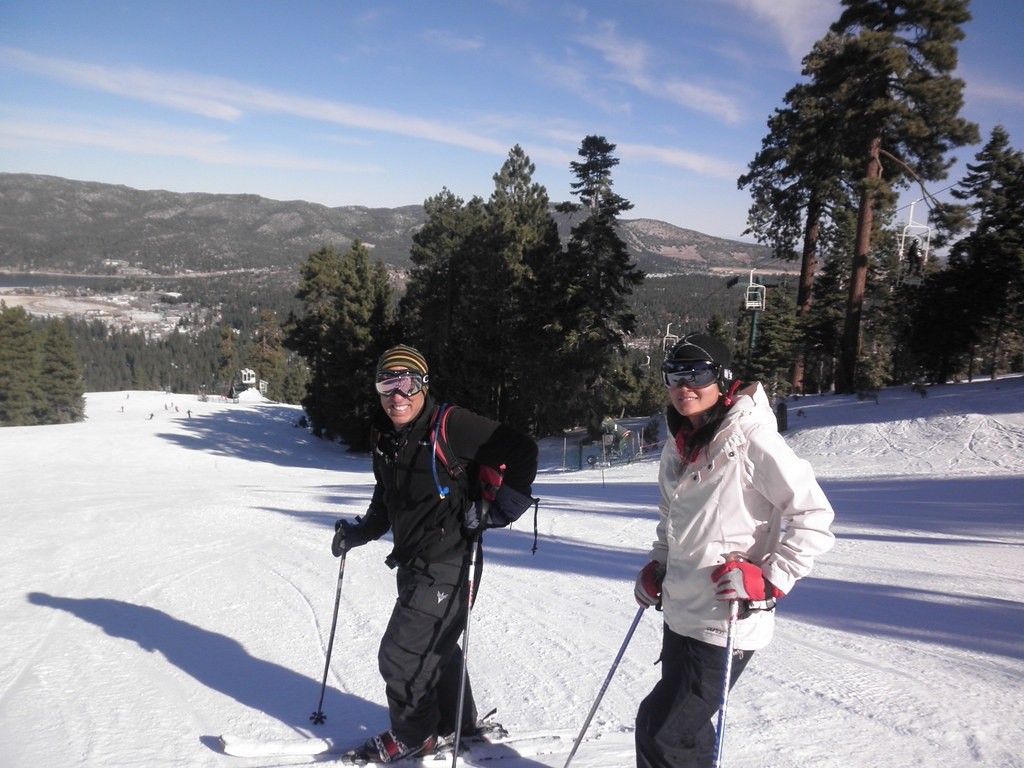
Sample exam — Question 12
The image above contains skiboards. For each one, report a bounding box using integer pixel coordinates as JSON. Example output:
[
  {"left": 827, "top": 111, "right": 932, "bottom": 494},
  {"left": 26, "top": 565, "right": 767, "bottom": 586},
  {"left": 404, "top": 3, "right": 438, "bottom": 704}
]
[{"left": 342, "top": 721, "right": 506, "bottom": 765}]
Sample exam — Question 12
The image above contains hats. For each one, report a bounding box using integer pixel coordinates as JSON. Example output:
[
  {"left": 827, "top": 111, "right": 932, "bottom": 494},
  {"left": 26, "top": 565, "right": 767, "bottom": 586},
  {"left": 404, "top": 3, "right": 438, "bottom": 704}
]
[
  {"left": 375, "top": 343, "right": 429, "bottom": 398},
  {"left": 668, "top": 332, "right": 732, "bottom": 398}
]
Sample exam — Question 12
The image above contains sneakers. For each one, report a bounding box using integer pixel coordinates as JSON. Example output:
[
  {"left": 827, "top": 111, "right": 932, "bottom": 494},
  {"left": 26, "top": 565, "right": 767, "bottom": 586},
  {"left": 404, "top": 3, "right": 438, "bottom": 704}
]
[
  {"left": 437, "top": 717, "right": 475, "bottom": 737},
  {"left": 364, "top": 729, "right": 437, "bottom": 762}
]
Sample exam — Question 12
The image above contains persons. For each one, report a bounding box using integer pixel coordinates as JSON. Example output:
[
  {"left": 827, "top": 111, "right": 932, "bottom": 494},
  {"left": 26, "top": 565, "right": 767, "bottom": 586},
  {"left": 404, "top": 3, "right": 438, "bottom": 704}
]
[
  {"left": 636, "top": 331, "right": 835, "bottom": 768},
  {"left": 120, "top": 394, "right": 193, "bottom": 419},
  {"left": 331, "top": 345, "right": 538, "bottom": 763},
  {"left": 298, "top": 415, "right": 310, "bottom": 431}
]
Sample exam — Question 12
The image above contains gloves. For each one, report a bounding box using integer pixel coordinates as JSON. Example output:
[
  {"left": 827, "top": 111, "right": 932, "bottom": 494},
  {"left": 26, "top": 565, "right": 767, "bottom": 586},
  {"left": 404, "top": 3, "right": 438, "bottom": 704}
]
[
  {"left": 483, "top": 505, "right": 507, "bottom": 530},
  {"left": 711, "top": 560, "right": 785, "bottom": 600},
  {"left": 332, "top": 516, "right": 370, "bottom": 557},
  {"left": 635, "top": 561, "right": 667, "bottom": 609}
]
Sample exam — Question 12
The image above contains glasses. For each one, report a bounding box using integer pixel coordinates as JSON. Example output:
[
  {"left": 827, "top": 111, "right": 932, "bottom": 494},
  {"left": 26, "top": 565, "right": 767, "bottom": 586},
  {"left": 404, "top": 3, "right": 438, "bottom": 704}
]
[
  {"left": 375, "top": 370, "right": 429, "bottom": 398},
  {"left": 661, "top": 361, "right": 734, "bottom": 390}
]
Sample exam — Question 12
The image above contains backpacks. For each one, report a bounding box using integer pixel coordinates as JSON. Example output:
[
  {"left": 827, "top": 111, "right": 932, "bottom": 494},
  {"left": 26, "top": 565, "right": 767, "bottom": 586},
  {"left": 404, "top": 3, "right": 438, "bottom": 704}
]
[{"left": 375, "top": 405, "right": 506, "bottom": 529}]
